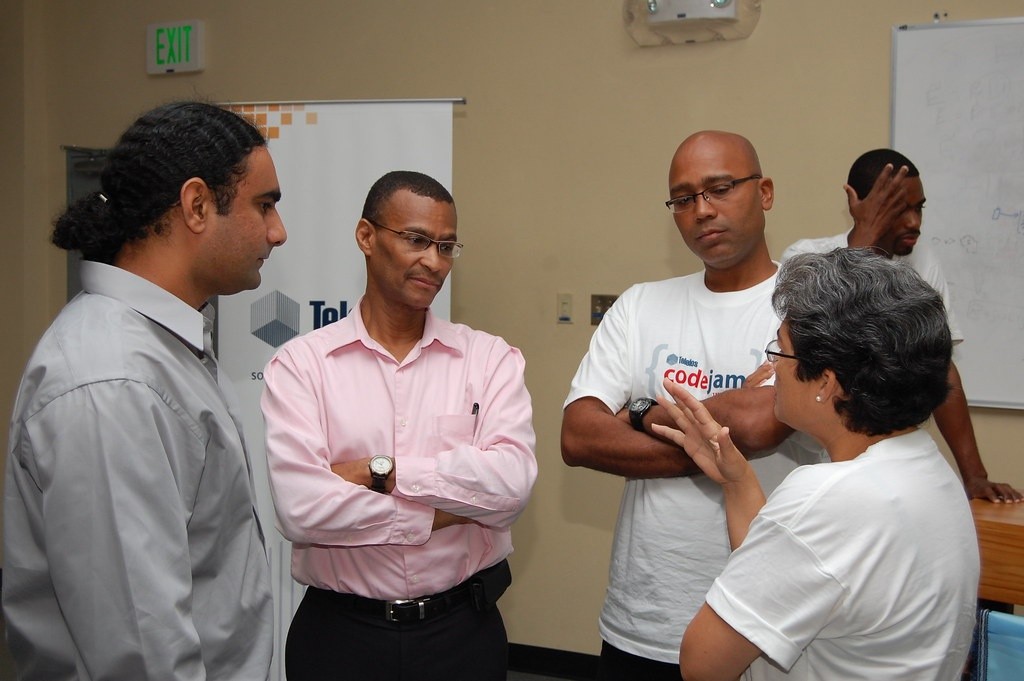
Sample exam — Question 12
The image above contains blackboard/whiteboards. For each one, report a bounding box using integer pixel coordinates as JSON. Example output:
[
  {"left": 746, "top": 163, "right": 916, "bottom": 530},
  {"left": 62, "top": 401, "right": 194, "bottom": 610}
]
[{"left": 887, "top": 18, "right": 1024, "bottom": 410}]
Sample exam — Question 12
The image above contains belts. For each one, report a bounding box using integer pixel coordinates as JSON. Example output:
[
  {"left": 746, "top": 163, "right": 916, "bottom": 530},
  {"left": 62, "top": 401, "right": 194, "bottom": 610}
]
[{"left": 310, "top": 579, "right": 477, "bottom": 623}]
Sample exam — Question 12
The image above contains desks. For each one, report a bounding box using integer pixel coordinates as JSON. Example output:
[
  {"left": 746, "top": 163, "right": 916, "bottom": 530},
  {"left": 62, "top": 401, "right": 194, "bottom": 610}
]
[{"left": 968, "top": 495, "right": 1024, "bottom": 616}]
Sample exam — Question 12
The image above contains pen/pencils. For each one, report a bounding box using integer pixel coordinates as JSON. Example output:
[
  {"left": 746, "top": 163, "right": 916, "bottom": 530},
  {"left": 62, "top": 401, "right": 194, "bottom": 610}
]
[{"left": 472, "top": 403, "right": 479, "bottom": 416}]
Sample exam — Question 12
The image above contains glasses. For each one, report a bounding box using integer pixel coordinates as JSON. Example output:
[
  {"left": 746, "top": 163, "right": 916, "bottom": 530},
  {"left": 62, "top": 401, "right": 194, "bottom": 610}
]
[
  {"left": 370, "top": 220, "right": 464, "bottom": 259},
  {"left": 665, "top": 175, "right": 762, "bottom": 214},
  {"left": 765, "top": 340, "right": 800, "bottom": 362}
]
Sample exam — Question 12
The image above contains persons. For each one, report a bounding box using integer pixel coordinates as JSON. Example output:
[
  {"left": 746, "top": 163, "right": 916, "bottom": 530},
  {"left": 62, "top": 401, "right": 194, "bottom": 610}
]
[
  {"left": 649, "top": 245, "right": 979, "bottom": 681},
  {"left": 777, "top": 147, "right": 1024, "bottom": 505},
  {"left": 263, "top": 169, "right": 537, "bottom": 681},
  {"left": 0, "top": 99, "right": 286, "bottom": 681},
  {"left": 558, "top": 132, "right": 828, "bottom": 680}
]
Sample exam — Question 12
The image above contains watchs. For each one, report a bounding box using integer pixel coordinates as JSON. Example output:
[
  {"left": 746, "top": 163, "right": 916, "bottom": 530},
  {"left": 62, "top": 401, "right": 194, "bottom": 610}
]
[
  {"left": 628, "top": 398, "right": 659, "bottom": 434},
  {"left": 367, "top": 454, "right": 394, "bottom": 493}
]
